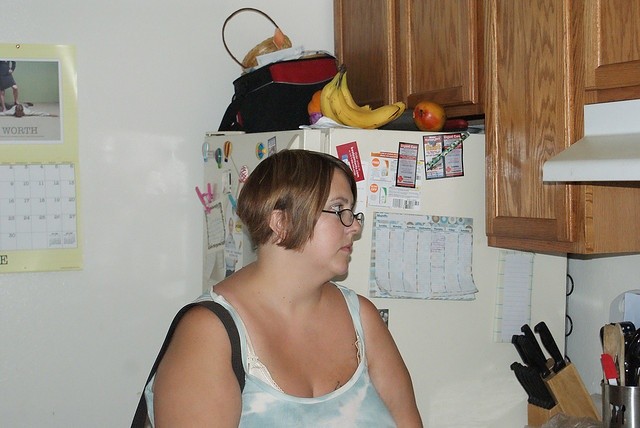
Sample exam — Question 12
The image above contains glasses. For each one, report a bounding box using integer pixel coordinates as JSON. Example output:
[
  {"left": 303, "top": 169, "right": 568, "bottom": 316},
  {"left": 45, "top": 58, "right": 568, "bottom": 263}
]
[{"left": 319, "top": 208, "right": 364, "bottom": 226}]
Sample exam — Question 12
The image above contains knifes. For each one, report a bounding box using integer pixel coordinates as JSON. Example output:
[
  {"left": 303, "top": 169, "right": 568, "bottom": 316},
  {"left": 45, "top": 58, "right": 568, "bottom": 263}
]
[{"left": 510, "top": 321, "right": 602, "bottom": 428}]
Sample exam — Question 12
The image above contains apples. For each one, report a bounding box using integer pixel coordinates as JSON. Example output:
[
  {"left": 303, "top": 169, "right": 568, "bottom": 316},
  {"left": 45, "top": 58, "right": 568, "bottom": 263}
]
[{"left": 413, "top": 101, "right": 446, "bottom": 130}]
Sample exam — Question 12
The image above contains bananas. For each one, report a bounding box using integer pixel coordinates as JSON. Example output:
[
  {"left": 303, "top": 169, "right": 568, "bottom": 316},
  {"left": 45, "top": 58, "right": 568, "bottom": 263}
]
[{"left": 321, "top": 64, "right": 405, "bottom": 129}]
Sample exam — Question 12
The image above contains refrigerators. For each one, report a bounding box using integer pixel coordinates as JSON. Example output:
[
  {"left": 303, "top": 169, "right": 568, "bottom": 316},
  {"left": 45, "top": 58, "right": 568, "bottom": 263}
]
[{"left": 201, "top": 131, "right": 567, "bottom": 428}]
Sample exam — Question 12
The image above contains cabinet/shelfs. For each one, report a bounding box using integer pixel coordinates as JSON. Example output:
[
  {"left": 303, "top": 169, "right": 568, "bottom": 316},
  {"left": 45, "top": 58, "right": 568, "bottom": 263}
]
[
  {"left": 584, "top": 0, "right": 640, "bottom": 105},
  {"left": 333, "top": 0, "right": 484, "bottom": 117},
  {"left": 484, "top": 0, "right": 640, "bottom": 255}
]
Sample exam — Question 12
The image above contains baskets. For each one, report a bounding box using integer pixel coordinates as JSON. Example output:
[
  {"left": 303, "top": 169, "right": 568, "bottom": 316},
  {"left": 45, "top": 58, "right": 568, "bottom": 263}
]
[{"left": 220, "top": 7, "right": 292, "bottom": 67}]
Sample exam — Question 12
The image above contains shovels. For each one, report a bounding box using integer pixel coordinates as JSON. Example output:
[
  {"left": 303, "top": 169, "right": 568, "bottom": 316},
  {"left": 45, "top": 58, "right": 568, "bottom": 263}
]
[
  {"left": 615, "top": 323, "right": 625, "bottom": 386},
  {"left": 603, "top": 323, "right": 620, "bottom": 384},
  {"left": 600, "top": 354, "right": 618, "bottom": 386},
  {"left": 618, "top": 322, "right": 636, "bottom": 385}
]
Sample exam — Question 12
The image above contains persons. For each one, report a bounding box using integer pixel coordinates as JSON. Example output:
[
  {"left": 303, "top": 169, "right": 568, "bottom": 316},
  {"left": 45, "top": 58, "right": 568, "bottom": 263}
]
[
  {"left": 0, "top": 61, "right": 20, "bottom": 112},
  {"left": 142, "top": 148, "right": 423, "bottom": 428}
]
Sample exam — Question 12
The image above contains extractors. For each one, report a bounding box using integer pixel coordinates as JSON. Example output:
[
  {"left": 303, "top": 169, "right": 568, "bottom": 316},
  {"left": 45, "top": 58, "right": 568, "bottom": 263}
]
[{"left": 542, "top": 99, "right": 640, "bottom": 182}]
